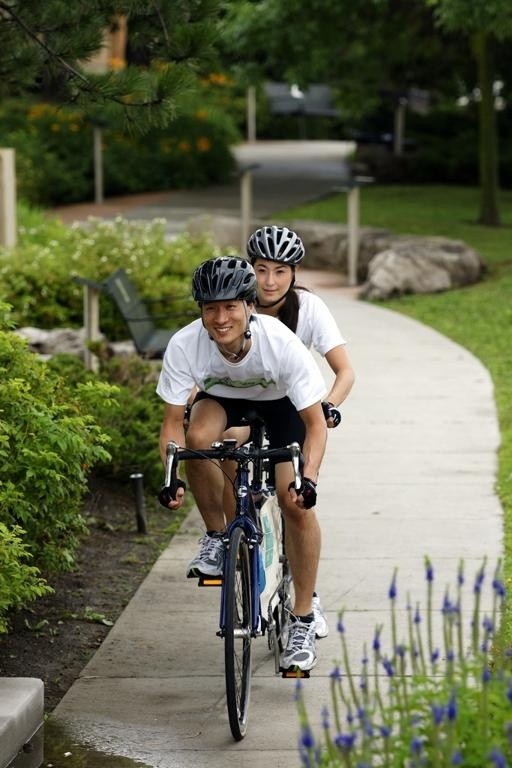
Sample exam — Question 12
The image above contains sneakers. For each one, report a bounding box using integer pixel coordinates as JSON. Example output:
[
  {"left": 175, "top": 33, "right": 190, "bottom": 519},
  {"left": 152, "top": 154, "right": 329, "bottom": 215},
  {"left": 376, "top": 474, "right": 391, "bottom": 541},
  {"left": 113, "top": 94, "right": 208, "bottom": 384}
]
[
  {"left": 186, "top": 535, "right": 225, "bottom": 577},
  {"left": 279, "top": 616, "right": 317, "bottom": 672},
  {"left": 313, "top": 594, "right": 329, "bottom": 638}
]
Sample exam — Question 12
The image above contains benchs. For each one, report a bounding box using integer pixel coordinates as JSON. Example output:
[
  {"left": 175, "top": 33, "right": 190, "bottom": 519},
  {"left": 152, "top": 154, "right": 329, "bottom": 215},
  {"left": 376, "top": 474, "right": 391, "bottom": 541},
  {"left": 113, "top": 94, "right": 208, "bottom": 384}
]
[{"left": 108, "top": 268, "right": 199, "bottom": 361}]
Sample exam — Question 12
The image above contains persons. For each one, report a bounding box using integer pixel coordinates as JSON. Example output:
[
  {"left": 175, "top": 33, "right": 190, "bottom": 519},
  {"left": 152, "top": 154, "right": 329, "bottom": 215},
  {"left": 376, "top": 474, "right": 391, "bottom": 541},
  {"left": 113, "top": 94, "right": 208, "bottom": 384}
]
[
  {"left": 221, "top": 226, "right": 355, "bottom": 641},
  {"left": 153, "top": 254, "right": 335, "bottom": 674}
]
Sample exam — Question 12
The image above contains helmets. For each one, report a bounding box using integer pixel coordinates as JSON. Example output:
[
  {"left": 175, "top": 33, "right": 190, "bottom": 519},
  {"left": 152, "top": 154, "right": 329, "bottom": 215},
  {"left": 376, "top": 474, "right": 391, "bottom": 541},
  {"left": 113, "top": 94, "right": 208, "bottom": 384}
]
[
  {"left": 192, "top": 256, "right": 256, "bottom": 301},
  {"left": 247, "top": 226, "right": 305, "bottom": 264}
]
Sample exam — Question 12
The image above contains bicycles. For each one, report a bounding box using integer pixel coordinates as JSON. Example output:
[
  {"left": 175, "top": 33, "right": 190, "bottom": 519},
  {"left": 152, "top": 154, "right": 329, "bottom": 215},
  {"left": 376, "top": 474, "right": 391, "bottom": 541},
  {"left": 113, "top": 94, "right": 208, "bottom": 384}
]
[{"left": 164, "top": 413, "right": 304, "bottom": 741}]
